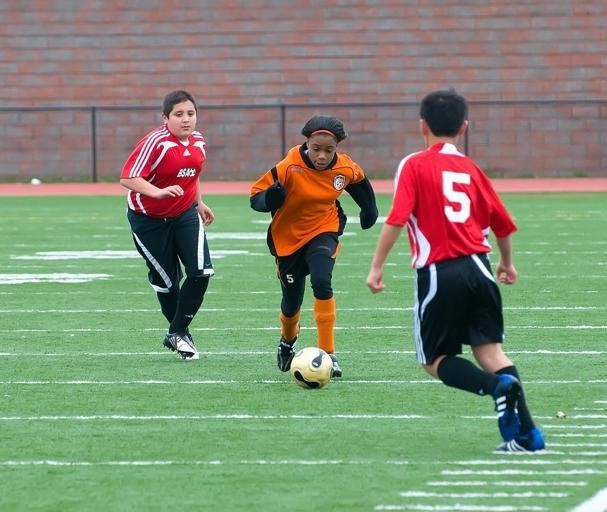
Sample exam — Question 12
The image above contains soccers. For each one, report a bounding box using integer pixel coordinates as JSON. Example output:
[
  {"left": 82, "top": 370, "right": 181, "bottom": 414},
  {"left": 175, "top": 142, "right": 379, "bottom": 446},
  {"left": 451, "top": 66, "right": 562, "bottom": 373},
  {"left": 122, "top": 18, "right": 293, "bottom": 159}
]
[
  {"left": 291, "top": 348, "right": 334, "bottom": 390},
  {"left": 29, "top": 178, "right": 42, "bottom": 185}
]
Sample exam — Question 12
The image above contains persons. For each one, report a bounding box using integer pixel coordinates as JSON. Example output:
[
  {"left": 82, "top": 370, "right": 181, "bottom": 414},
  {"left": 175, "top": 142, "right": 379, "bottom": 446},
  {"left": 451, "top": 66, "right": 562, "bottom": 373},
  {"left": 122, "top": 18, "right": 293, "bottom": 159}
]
[
  {"left": 249, "top": 114, "right": 379, "bottom": 377},
  {"left": 119, "top": 90, "right": 216, "bottom": 359},
  {"left": 366, "top": 90, "right": 545, "bottom": 453}
]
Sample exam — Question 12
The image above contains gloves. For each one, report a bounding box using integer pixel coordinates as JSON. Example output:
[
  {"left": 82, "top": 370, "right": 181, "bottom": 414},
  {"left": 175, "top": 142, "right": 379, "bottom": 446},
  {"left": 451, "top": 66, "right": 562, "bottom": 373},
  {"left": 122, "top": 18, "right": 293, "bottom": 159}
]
[
  {"left": 264, "top": 181, "right": 285, "bottom": 210},
  {"left": 359, "top": 206, "right": 378, "bottom": 229}
]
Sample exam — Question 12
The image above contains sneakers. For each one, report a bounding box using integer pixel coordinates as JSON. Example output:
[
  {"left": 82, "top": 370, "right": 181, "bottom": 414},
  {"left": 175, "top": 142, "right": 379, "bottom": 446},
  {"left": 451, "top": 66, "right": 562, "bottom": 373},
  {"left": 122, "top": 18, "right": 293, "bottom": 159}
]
[
  {"left": 492, "top": 374, "right": 545, "bottom": 455},
  {"left": 327, "top": 354, "right": 342, "bottom": 377},
  {"left": 277, "top": 322, "right": 300, "bottom": 372},
  {"left": 162, "top": 327, "right": 200, "bottom": 360}
]
